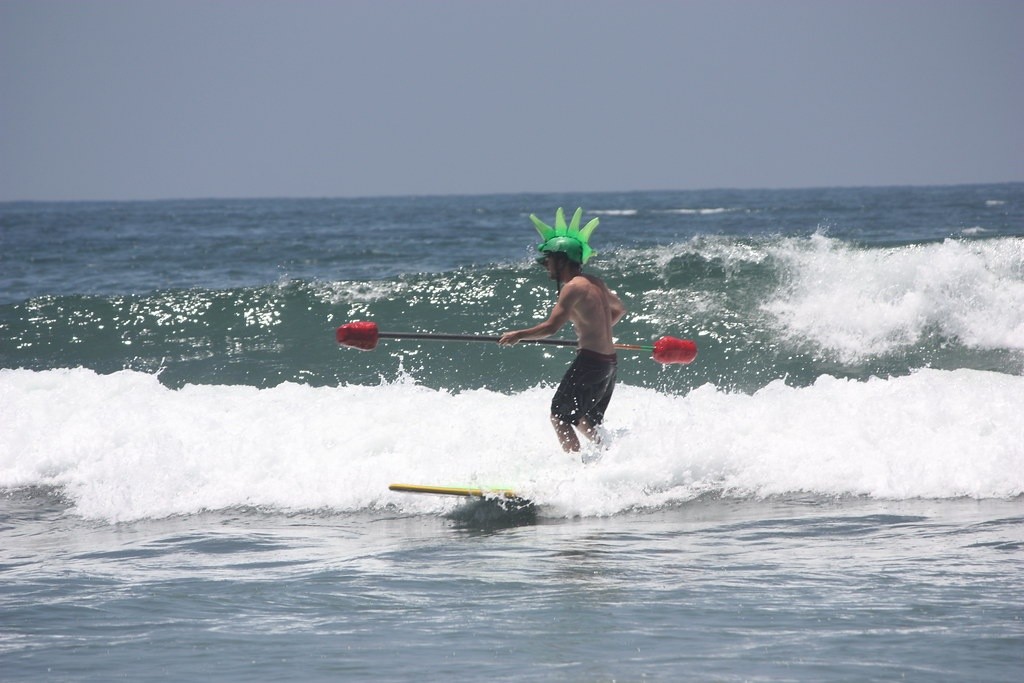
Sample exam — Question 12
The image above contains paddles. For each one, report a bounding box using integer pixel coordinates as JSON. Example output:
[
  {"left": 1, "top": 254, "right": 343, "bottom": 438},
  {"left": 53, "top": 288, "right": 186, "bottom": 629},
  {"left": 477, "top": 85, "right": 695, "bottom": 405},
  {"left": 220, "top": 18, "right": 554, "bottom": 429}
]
[{"left": 335, "top": 320, "right": 699, "bottom": 365}]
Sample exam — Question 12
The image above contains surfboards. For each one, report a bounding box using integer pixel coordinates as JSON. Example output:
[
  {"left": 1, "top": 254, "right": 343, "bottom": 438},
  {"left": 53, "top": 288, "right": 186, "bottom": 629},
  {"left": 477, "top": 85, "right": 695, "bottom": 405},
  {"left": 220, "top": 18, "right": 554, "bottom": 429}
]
[{"left": 388, "top": 482, "right": 519, "bottom": 499}]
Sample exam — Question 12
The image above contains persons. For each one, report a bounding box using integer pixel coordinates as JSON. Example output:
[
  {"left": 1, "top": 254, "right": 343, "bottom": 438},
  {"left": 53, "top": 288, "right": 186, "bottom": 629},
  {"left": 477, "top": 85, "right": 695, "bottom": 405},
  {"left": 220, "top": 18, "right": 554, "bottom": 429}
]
[{"left": 498, "top": 236, "right": 627, "bottom": 452}]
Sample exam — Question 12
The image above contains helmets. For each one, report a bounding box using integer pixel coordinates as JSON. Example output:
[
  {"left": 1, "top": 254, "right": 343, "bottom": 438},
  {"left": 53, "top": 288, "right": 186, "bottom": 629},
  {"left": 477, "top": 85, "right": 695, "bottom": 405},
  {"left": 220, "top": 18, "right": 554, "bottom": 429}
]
[{"left": 529, "top": 206, "right": 599, "bottom": 264}]
